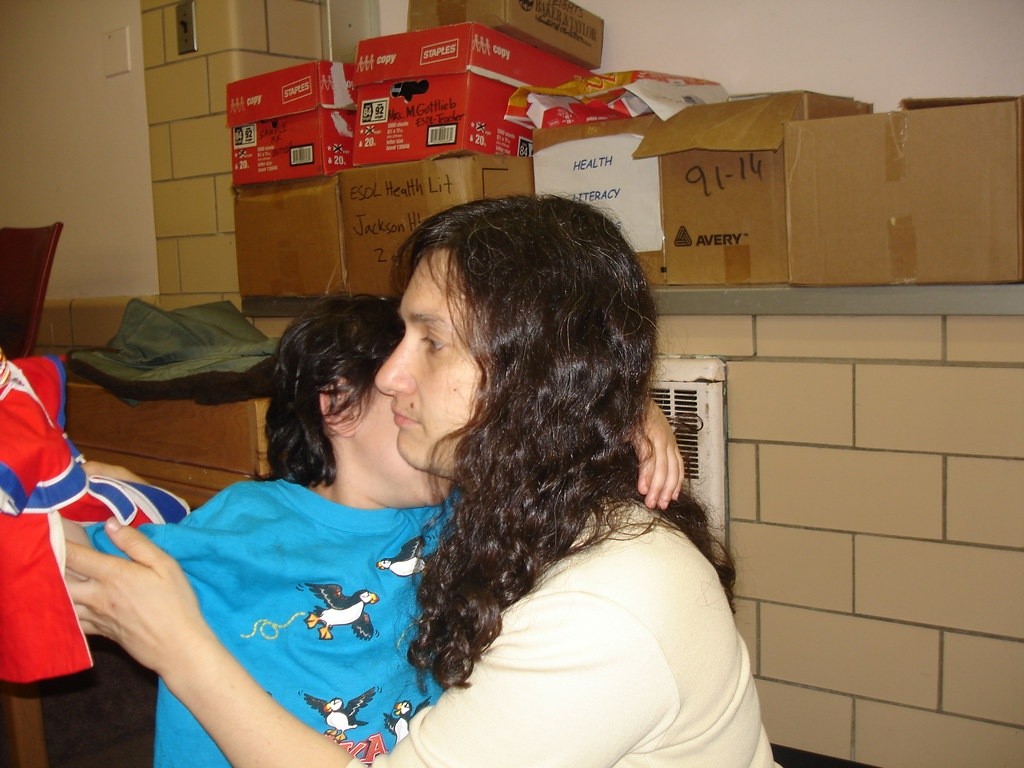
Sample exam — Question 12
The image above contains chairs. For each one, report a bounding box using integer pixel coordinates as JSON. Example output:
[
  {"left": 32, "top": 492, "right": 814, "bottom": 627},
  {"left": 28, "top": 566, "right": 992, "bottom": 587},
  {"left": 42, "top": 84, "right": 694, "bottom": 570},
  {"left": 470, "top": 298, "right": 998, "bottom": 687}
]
[{"left": 0, "top": 221, "right": 63, "bottom": 768}]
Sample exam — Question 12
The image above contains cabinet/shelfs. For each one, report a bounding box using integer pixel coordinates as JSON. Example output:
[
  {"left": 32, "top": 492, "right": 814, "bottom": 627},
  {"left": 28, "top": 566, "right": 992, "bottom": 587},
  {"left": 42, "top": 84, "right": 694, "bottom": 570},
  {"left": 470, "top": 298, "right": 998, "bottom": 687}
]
[{"left": 64, "top": 365, "right": 274, "bottom": 514}]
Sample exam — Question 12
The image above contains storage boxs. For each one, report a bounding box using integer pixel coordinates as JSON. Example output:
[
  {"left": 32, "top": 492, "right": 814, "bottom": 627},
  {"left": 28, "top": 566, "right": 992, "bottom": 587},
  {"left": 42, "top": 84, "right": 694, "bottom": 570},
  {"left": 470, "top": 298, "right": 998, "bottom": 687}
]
[{"left": 229, "top": 0, "right": 1024, "bottom": 299}]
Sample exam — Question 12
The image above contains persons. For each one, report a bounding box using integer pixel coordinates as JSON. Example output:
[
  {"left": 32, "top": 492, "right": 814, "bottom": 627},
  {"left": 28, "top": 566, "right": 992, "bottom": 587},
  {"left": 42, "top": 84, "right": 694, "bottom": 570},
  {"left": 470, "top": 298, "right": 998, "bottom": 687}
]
[
  {"left": 64, "top": 196, "right": 789, "bottom": 768},
  {"left": 64, "top": 294, "right": 687, "bottom": 768}
]
